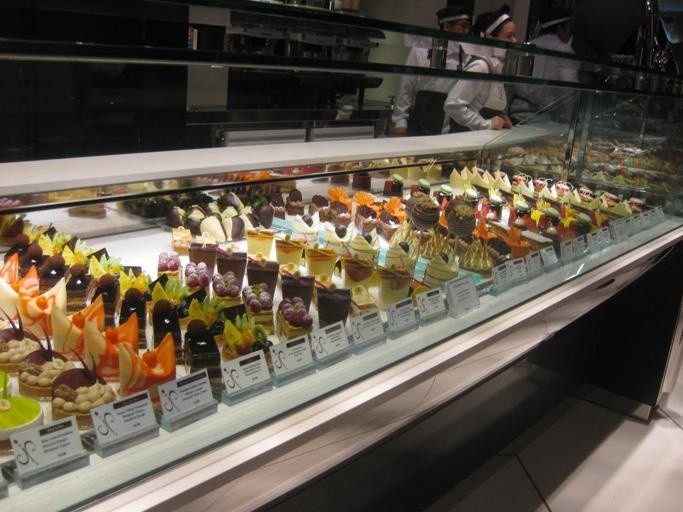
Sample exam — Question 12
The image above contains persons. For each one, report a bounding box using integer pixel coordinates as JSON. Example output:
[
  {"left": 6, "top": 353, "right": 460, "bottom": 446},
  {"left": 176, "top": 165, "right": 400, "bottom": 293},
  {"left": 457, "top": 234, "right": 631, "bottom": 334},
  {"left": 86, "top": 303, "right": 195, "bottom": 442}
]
[
  {"left": 438, "top": 3, "right": 524, "bottom": 131},
  {"left": 390, "top": 1, "right": 485, "bottom": 138},
  {"left": 505, "top": 7, "right": 582, "bottom": 126},
  {"left": 567, "top": 29, "right": 621, "bottom": 87}
]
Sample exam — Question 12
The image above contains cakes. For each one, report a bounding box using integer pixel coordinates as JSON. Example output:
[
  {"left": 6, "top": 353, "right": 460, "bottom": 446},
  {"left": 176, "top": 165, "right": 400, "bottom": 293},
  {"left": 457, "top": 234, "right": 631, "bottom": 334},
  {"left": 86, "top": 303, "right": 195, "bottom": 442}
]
[{"left": 502, "top": 138, "right": 680, "bottom": 195}]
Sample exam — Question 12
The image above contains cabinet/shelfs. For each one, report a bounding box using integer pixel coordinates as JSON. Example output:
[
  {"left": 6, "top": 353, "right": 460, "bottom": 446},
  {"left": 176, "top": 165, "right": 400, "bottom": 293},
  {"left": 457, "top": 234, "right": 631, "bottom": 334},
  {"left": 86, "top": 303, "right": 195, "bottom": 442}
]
[{"left": 0, "top": 0, "right": 683, "bottom": 512}]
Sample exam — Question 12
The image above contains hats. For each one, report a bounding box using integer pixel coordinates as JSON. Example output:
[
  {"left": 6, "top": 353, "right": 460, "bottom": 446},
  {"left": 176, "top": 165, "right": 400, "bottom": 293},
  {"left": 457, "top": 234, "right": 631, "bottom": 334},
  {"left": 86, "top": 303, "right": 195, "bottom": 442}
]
[
  {"left": 470, "top": 3, "right": 512, "bottom": 39},
  {"left": 538, "top": 7, "right": 570, "bottom": 29},
  {"left": 436, "top": 3, "right": 473, "bottom": 25}
]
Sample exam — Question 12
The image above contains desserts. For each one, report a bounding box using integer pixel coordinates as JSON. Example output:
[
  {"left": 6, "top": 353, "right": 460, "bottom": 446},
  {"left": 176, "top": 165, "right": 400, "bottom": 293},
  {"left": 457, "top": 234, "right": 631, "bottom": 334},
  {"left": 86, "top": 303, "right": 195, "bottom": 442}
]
[{"left": 1, "top": 160, "right": 645, "bottom": 440}]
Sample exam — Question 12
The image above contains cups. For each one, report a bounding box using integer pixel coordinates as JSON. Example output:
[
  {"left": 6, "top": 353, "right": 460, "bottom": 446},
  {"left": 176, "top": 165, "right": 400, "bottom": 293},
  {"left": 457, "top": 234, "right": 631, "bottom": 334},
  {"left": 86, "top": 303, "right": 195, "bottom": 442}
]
[{"left": 507, "top": 42, "right": 535, "bottom": 74}]
